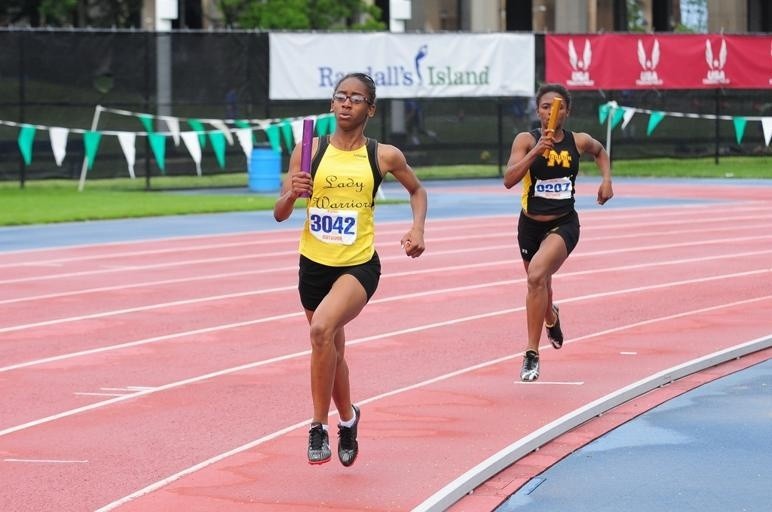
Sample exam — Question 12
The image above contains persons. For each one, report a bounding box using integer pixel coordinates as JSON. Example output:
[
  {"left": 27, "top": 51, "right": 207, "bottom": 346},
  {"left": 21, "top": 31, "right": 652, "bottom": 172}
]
[
  {"left": 272, "top": 70, "right": 429, "bottom": 467},
  {"left": 503, "top": 81, "right": 614, "bottom": 383}
]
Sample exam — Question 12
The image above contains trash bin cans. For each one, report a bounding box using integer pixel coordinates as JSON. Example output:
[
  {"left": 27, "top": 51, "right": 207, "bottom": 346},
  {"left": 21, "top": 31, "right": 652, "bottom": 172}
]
[{"left": 249, "top": 144, "right": 282, "bottom": 193}]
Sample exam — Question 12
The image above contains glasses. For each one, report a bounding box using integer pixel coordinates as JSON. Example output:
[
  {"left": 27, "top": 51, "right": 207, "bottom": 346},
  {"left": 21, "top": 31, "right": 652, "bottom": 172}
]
[{"left": 333, "top": 92, "right": 372, "bottom": 105}]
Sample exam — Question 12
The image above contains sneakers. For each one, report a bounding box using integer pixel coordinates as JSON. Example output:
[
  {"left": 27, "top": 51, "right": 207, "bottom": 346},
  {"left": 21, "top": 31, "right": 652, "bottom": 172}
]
[
  {"left": 544, "top": 304, "right": 564, "bottom": 349},
  {"left": 307, "top": 424, "right": 332, "bottom": 465},
  {"left": 337, "top": 404, "right": 361, "bottom": 467},
  {"left": 520, "top": 350, "right": 540, "bottom": 382}
]
